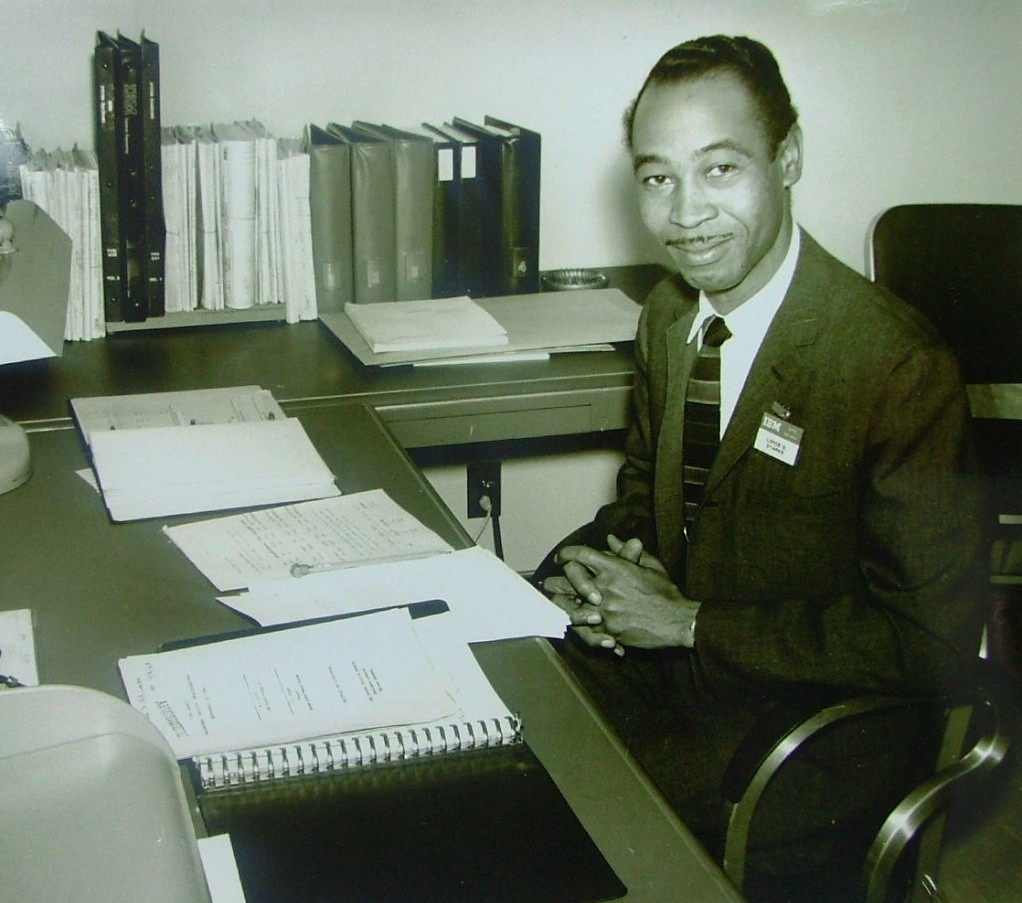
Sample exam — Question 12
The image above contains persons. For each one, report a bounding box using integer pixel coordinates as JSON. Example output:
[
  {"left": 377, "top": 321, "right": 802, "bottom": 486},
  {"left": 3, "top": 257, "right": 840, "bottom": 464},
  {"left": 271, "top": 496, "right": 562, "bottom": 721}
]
[{"left": 528, "top": 35, "right": 995, "bottom": 851}]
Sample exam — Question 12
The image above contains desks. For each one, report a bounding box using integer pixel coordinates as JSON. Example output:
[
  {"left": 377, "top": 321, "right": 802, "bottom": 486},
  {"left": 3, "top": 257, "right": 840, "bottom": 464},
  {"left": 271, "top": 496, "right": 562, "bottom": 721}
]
[
  {"left": 0, "top": 403, "right": 747, "bottom": 903},
  {"left": 1, "top": 262, "right": 673, "bottom": 448}
]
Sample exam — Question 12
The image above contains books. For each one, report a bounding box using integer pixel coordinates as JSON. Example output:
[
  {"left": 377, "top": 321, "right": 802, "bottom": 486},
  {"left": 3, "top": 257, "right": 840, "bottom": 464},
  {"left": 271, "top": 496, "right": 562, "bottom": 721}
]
[{"left": 0, "top": 29, "right": 648, "bottom": 795}]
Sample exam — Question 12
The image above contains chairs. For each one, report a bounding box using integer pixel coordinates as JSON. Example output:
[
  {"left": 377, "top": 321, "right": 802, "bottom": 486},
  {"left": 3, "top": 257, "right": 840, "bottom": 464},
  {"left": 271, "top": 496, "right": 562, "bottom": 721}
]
[{"left": 533, "top": 203, "right": 1022, "bottom": 903}]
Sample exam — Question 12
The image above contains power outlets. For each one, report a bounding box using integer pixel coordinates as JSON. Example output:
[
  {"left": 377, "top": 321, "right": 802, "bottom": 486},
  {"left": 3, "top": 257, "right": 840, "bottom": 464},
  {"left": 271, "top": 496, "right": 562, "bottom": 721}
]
[{"left": 467, "top": 460, "right": 501, "bottom": 519}]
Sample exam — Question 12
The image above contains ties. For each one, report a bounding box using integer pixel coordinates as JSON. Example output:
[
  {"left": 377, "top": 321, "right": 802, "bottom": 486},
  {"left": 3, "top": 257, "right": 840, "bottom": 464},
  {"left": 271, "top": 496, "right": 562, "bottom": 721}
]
[{"left": 682, "top": 314, "right": 732, "bottom": 553}]
